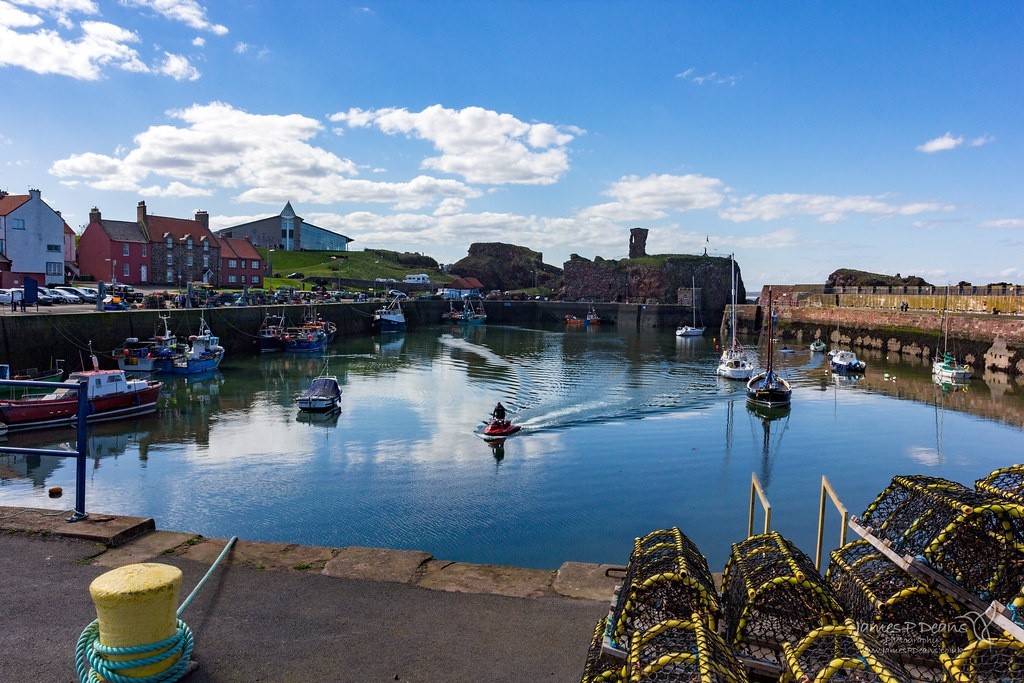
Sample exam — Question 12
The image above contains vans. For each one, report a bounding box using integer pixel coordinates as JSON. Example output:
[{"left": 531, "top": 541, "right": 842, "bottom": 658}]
[{"left": 436, "top": 292, "right": 450, "bottom": 300}]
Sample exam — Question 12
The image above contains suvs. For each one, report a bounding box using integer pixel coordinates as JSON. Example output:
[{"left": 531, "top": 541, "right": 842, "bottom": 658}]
[
  {"left": 105, "top": 284, "right": 144, "bottom": 303},
  {"left": 461, "top": 293, "right": 478, "bottom": 300}
]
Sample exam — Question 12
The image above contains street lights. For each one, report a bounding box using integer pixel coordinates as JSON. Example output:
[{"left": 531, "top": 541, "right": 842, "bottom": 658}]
[
  {"left": 268, "top": 247, "right": 275, "bottom": 291},
  {"left": 339, "top": 261, "right": 343, "bottom": 290},
  {"left": 828, "top": 310, "right": 843, "bottom": 359}
]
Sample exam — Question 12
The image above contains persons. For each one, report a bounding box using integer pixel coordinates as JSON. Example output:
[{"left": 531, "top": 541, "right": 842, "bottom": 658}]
[
  {"left": 492, "top": 402, "right": 505, "bottom": 426},
  {"left": 175, "top": 295, "right": 179, "bottom": 309},
  {"left": 900, "top": 301, "right": 909, "bottom": 311},
  {"left": 353, "top": 293, "right": 367, "bottom": 301}
]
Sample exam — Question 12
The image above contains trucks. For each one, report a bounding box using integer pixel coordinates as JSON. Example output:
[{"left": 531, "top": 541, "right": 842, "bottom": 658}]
[{"left": 403, "top": 275, "right": 429, "bottom": 284}]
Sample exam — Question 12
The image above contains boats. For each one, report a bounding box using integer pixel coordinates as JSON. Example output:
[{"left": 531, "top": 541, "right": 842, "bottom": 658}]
[
  {"left": 0, "top": 365, "right": 64, "bottom": 399},
  {"left": 0, "top": 369, "right": 163, "bottom": 433},
  {"left": 371, "top": 304, "right": 406, "bottom": 334},
  {"left": 565, "top": 313, "right": 602, "bottom": 327},
  {"left": 828, "top": 348, "right": 866, "bottom": 375},
  {"left": 152, "top": 317, "right": 225, "bottom": 373},
  {"left": 112, "top": 313, "right": 190, "bottom": 373},
  {"left": 810, "top": 340, "right": 826, "bottom": 352},
  {"left": 278, "top": 311, "right": 337, "bottom": 352},
  {"left": 256, "top": 314, "right": 300, "bottom": 353},
  {"left": 441, "top": 300, "right": 487, "bottom": 325},
  {"left": 294, "top": 360, "right": 343, "bottom": 409},
  {"left": 778, "top": 349, "right": 796, "bottom": 353}
]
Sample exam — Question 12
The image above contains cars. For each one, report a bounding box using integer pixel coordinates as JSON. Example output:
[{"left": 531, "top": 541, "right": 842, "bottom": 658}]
[
  {"left": 233, "top": 290, "right": 345, "bottom": 298},
  {"left": 387, "top": 279, "right": 399, "bottom": 282},
  {"left": 389, "top": 290, "right": 407, "bottom": 298},
  {"left": 286, "top": 273, "right": 305, "bottom": 279},
  {"left": 0, "top": 286, "right": 110, "bottom": 305},
  {"left": 479, "top": 294, "right": 512, "bottom": 301}
]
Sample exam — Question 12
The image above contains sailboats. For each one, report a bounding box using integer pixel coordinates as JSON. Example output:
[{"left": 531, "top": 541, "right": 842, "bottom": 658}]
[
  {"left": 746, "top": 286, "right": 792, "bottom": 408},
  {"left": 676, "top": 275, "right": 706, "bottom": 336},
  {"left": 932, "top": 282, "right": 975, "bottom": 379},
  {"left": 716, "top": 251, "right": 754, "bottom": 379}
]
